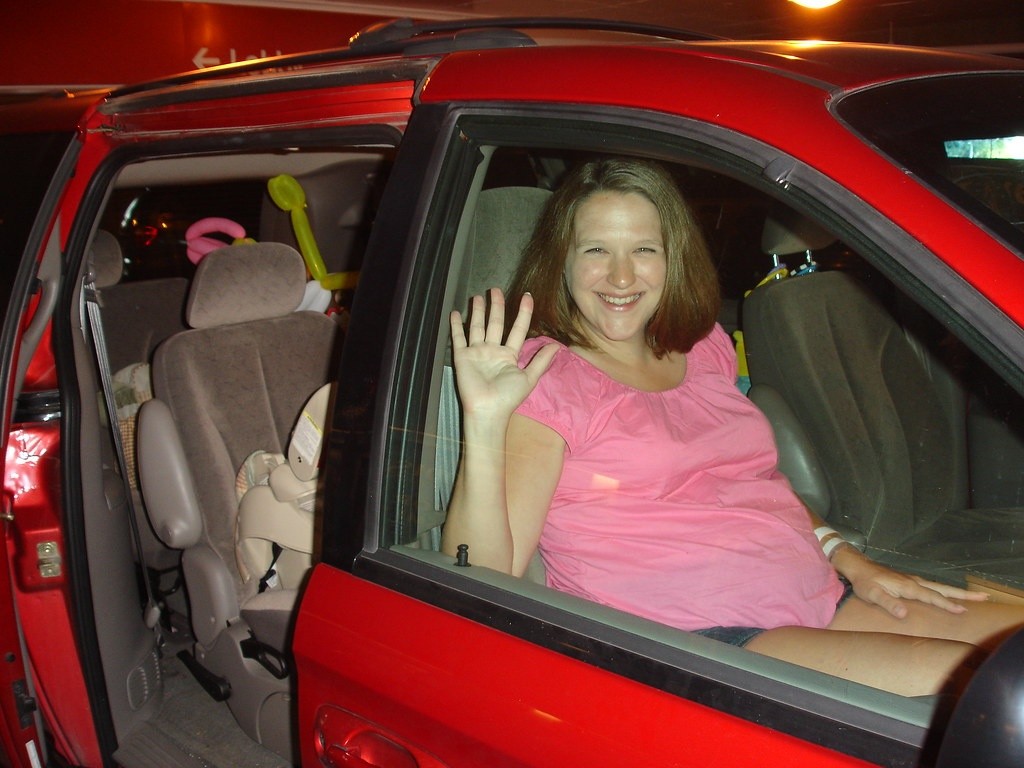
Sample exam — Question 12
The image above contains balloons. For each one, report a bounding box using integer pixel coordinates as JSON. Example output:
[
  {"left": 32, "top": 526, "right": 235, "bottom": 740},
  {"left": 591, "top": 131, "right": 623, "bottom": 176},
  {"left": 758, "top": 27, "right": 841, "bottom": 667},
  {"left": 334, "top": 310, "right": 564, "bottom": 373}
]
[{"left": 179, "top": 175, "right": 361, "bottom": 312}]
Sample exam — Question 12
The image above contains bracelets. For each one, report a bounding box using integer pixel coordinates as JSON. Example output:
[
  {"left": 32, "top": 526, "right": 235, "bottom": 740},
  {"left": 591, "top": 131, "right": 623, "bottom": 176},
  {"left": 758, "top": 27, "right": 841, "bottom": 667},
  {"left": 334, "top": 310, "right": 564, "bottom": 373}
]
[{"left": 813, "top": 526, "right": 854, "bottom": 558}]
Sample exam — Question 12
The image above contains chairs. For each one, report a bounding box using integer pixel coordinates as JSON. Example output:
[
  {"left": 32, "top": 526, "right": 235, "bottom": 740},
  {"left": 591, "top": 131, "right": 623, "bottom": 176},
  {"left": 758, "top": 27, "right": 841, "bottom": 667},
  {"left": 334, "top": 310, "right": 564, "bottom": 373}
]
[{"left": 92, "top": 161, "right": 1024, "bottom": 761}]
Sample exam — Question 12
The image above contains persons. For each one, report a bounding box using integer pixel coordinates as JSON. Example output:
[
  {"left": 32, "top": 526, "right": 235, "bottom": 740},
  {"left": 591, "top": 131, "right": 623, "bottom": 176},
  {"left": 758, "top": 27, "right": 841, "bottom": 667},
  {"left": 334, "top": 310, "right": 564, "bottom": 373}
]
[{"left": 437, "top": 159, "right": 1024, "bottom": 699}]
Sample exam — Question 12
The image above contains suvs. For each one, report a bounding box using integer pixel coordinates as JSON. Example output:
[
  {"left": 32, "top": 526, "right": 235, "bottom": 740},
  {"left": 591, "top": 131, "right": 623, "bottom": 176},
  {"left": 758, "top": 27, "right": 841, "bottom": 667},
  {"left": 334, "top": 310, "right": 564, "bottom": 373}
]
[{"left": 0, "top": 12, "right": 1024, "bottom": 767}]
